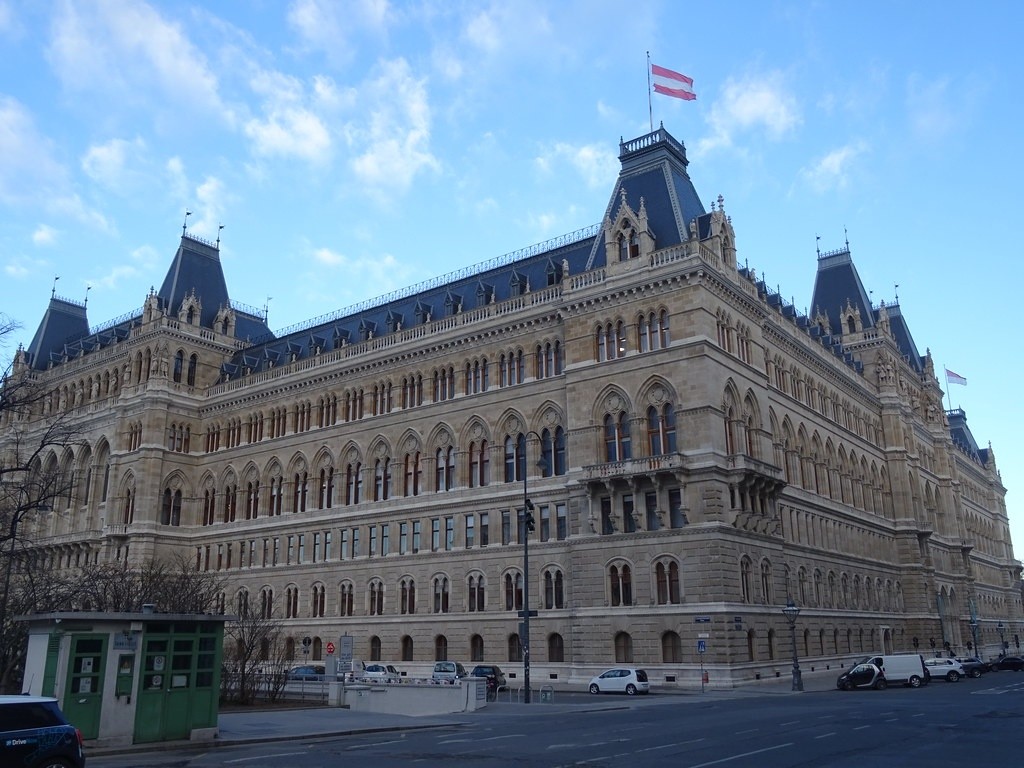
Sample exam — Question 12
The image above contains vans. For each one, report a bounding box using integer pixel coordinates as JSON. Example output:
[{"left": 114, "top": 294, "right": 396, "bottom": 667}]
[
  {"left": 432, "top": 661, "right": 470, "bottom": 684},
  {"left": 364, "top": 664, "right": 404, "bottom": 684},
  {"left": 859, "top": 653, "right": 925, "bottom": 687}
]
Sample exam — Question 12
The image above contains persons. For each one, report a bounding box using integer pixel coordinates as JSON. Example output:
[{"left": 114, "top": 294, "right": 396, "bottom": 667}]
[{"left": 998, "top": 653, "right": 1003, "bottom": 660}]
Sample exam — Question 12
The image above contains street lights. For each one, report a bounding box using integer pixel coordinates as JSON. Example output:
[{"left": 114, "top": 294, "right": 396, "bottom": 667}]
[
  {"left": 781, "top": 591, "right": 804, "bottom": 690},
  {"left": 967, "top": 616, "right": 979, "bottom": 658},
  {"left": 995, "top": 618, "right": 1006, "bottom": 656}
]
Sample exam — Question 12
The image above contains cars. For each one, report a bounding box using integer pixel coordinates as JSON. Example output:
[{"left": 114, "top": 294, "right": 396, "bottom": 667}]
[
  {"left": 588, "top": 668, "right": 650, "bottom": 695},
  {"left": 285, "top": 664, "right": 326, "bottom": 680},
  {"left": 985, "top": 656, "right": 1024, "bottom": 672},
  {"left": 952, "top": 655, "right": 986, "bottom": 679},
  {"left": 924, "top": 658, "right": 966, "bottom": 682},
  {"left": 836, "top": 664, "right": 888, "bottom": 690},
  {"left": 470, "top": 664, "right": 507, "bottom": 691},
  {"left": 0, "top": 690, "right": 88, "bottom": 768}
]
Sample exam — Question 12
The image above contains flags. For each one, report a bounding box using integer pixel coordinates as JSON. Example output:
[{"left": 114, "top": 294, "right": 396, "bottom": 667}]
[
  {"left": 652, "top": 64, "right": 698, "bottom": 104},
  {"left": 946, "top": 369, "right": 968, "bottom": 387}
]
